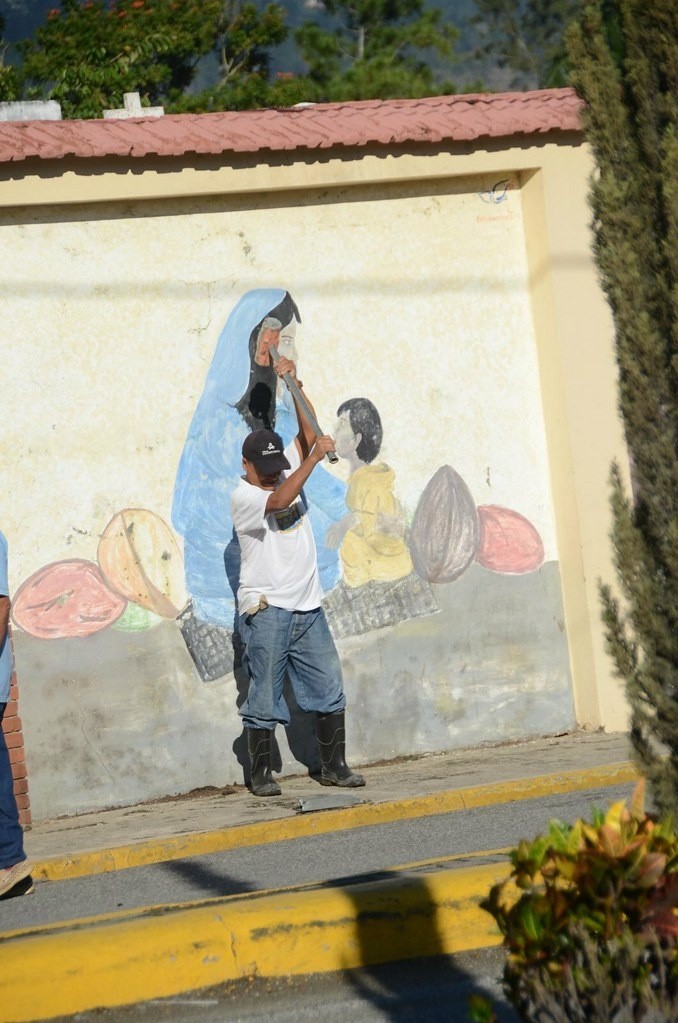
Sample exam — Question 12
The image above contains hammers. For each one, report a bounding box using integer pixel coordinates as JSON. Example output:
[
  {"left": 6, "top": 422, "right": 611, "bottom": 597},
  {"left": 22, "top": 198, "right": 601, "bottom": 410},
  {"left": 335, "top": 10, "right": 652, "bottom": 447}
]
[{"left": 253, "top": 316, "right": 339, "bottom": 464}]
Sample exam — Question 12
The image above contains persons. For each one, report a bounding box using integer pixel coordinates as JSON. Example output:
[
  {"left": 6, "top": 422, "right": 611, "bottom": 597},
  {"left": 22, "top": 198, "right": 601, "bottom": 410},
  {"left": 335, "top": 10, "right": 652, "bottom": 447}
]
[{"left": 233, "top": 355, "right": 366, "bottom": 798}]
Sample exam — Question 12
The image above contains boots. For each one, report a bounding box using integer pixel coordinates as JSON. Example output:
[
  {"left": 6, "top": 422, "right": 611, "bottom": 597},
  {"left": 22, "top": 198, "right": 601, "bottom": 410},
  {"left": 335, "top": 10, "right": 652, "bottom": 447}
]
[
  {"left": 313, "top": 711, "right": 369, "bottom": 787},
  {"left": 246, "top": 727, "right": 282, "bottom": 796}
]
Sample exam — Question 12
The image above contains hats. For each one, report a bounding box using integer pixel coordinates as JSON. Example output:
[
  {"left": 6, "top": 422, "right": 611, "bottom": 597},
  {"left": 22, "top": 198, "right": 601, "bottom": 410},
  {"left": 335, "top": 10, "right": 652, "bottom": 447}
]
[{"left": 242, "top": 430, "right": 291, "bottom": 476}]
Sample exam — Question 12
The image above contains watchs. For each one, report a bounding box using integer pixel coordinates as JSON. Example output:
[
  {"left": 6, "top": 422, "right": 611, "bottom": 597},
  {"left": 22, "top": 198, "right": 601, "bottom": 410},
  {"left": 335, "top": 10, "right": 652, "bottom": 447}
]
[{"left": 287, "top": 381, "right": 303, "bottom": 391}]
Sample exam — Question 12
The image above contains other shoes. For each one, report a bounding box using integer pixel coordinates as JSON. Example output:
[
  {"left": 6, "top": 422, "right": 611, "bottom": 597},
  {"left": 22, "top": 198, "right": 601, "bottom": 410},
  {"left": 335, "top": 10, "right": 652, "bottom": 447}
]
[{"left": 1, "top": 859, "right": 34, "bottom": 895}]
